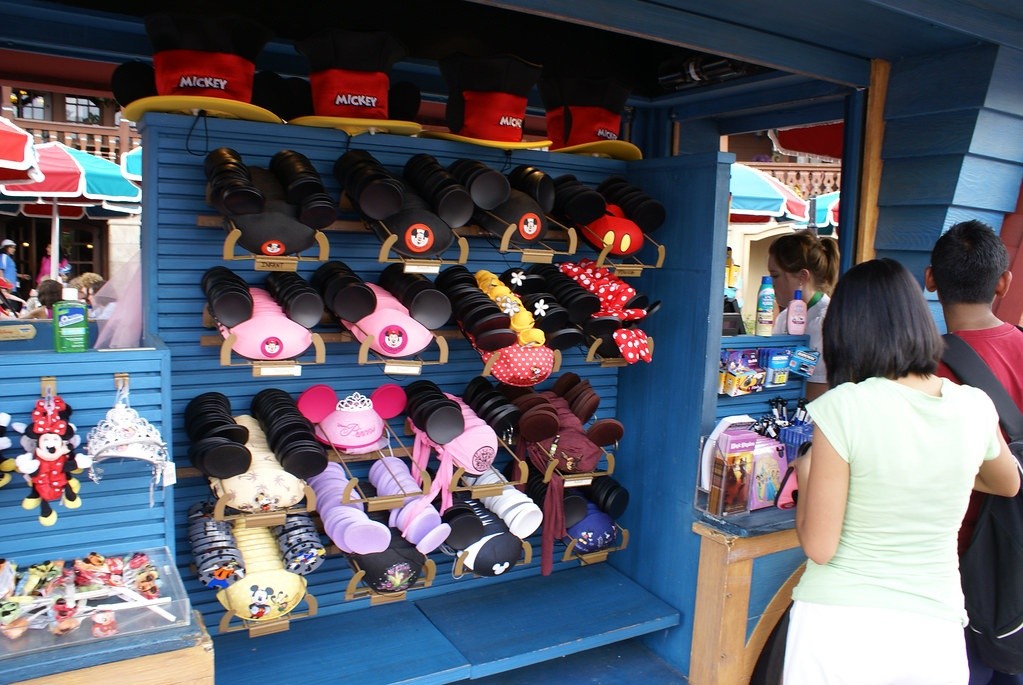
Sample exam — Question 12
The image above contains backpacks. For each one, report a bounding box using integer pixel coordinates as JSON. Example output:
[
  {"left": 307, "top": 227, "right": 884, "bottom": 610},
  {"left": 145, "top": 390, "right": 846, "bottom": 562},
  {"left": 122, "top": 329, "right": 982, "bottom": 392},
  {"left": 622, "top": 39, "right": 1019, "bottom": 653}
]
[{"left": 937, "top": 325, "right": 1023, "bottom": 674}]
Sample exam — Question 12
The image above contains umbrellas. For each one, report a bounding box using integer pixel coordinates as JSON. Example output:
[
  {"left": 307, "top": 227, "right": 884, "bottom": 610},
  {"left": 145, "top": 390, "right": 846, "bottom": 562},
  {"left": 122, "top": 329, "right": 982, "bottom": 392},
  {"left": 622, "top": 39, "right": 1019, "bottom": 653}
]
[
  {"left": 122, "top": 145, "right": 143, "bottom": 183},
  {"left": 768, "top": 119, "right": 845, "bottom": 161},
  {"left": 730, "top": 162, "right": 808, "bottom": 225},
  {"left": 792, "top": 190, "right": 839, "bottom": 236},
  {"left": 0, "top": 141, "right": 143, "bottom": 278},
  {"left": 0, "top": 115, "right": 45, "bottom": 184}
]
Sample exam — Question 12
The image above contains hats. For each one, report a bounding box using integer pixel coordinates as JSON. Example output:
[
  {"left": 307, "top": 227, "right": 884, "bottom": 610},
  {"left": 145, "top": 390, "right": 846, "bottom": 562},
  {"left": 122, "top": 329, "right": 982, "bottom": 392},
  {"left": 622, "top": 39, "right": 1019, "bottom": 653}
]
[
  {"left": 542, "top": 108, "right": 642, "bottom": 159},
  {"left": 0, "top": 239, "right": 17, "bottom": 249},
  {"left": 0, "top": 278, "right": 14, "bottom": 289},
  {"left": 120, "top": 49, "right": 284, "bottom": 123},
  {"left": 424, "top": 89, "right": 553, "bottom": 150},
  {"left": 173, "top": 148, "right": 654, "bottom": 628},
  {"left": 287, "top": 69, "right": 422, "bottom": 135}
]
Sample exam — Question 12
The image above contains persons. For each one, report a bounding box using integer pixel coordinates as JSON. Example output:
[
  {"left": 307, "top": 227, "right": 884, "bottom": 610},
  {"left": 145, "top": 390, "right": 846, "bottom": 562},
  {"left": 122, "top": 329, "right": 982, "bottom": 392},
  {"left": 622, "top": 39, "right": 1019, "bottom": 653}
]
[
  {"left": 924, "top": 220, "right": 1023, "bottom": 685},
  {"left": 0, "top": 239, "right": 117, "bottom": 321},
  {"left": 766, "top": 232, "right": 840, "bottom": 403},
  {"left": 781, "top": 259, "right": 1023, "bottom": 685}
]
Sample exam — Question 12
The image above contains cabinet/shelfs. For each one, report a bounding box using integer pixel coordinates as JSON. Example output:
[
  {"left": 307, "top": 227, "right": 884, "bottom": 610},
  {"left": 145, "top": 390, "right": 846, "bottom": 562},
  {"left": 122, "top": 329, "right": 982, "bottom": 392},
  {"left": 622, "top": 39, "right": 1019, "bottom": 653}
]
[{"left": 0, "top": 113, "right": 812, "bottom": 685}]
